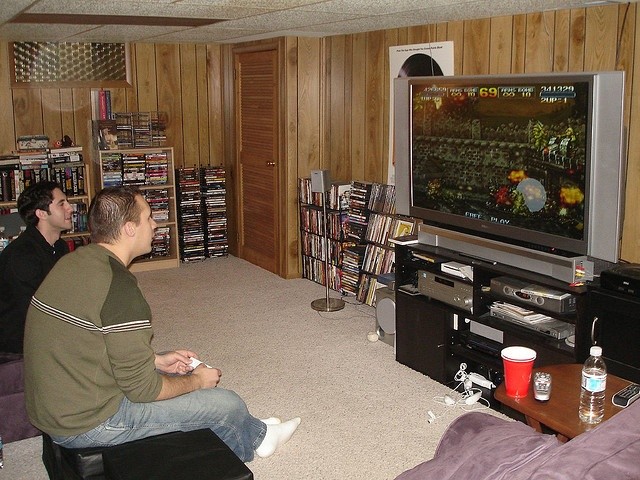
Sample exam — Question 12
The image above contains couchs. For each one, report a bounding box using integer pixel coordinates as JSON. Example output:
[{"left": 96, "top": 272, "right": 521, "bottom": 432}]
[
  {"left": 393, "top": 398, "right": 640, "bottom": 480},
  {"left": 1, "top": 349, "right": 45, "bottom": 447}
]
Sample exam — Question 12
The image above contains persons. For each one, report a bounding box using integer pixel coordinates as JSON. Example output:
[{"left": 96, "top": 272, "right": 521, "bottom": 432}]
[
  {"left": 23, "top": 187, "right": 301, "bottom": 464},
  {"left": 1, "top": 180, "right": 73, "bottom": 354}
]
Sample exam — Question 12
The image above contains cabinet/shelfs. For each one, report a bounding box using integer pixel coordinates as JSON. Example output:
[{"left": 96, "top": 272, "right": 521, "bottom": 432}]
[
  {"left": 341, "top": 181, "right": 422, "bottom": 309},
  {"left": 175, "top": 165, "right": 206, "bottom": 262},
  {"left": 92, "top": 150, "right": 180, "bottom": 272},
  {"left": 395, "top": 238, "right": 639, "bottom": 435},
  {"left": 89, "top": 112, "right": 166, "bottom": 149},
  {"left": 0, "top": 160, "right": 94, "bottom": 253},
  {"left": 201, "top": 163, "right": 228, "bottom": 260},
  {"left": 298, "top": 177, "right": 350, "bottom": 294}
]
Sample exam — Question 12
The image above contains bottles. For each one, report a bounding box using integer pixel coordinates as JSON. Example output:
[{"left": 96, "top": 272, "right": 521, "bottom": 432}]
[{"left": 578, "top": 345, "right": 607, "bottom": 425}]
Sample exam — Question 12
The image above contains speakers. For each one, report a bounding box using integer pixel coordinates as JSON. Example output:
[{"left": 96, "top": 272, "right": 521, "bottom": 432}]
[
  {"left": 373, "top": 287, "right": 396, "bottom": 348},
  {"left": 310, "top": 168, "right": 331, "bottom": 194}
]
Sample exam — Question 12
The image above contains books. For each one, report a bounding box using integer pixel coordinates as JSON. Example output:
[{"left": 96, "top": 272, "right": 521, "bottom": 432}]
[
  {"left": 349, "top": 179, "right": 371, "bottom": 206},
  {"left": 365, "top": 213, "right": 414, "bottom": 249},
  {"left": 1, "top": 205, "right": 16, "bottom": 239},
  {"left": 200, "top": 166, "right": 229, "bottom": 259},
  {"left": 299, "top": 231, "right": 343, "bottom": 267},
  {"left": 346, "top": 209, "right": 369, "bottom": 240},
  {"left": 63, "top": 202, "right": 88, "bottom": 232},
  {"left": 369, "top": 181, "right": 397, "bottom": 214},
  {"left": 300, "top": 179, "right": 351, "bottom": 210},
  {"left": 140, "top": 189, "right": 169, "bottom": 221},
  {"left": 1, "top": 135, "right": 87, "bottom": 204},
  {"left": 91, "top": 90, "right": 111, "bottom": 121},
  {"left": 489, "top": 301, "right": 553, "bottom": 324},
  {"left": 358, "top": 274, "right": 387, "bottom": 309},
  {"left": 100, "top": 152, "right": 169, "bottom": 188},
  {"left": 300, "top": 205, "right": 349, "bottom": 241},
  {"left": 174, "top": 168, "right": 205, "bottom": 263},
  {"left": 65, "top": 235, "right": 89, "bottom": 252},
  {"left": 360, "top": 243, "right": 396, "bottom": 277},
  {"left": 136, "top": 228, "right": 171, "bottom": 260},
  {"left": 341, "top": 246, "right": 363, "bottom": 296},
  {"left": 301, "top": 255, "right": 342, "bottom": 293},
  {"left": 98, "top": 122, "right": 118, "bottom": 149}
]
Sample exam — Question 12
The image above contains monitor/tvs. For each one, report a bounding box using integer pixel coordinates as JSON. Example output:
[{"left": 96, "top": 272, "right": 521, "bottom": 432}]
[{"left": 391, "top": 69, "right": 627, "bottom": 284}]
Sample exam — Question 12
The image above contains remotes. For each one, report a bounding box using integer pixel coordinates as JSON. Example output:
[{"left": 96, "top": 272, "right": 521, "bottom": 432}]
[
  {"left": 533, "top": 371, "right": 552, "bottom": 402},
  {"left": 610, "top": 381, "right": 640, "bottom": 410}
]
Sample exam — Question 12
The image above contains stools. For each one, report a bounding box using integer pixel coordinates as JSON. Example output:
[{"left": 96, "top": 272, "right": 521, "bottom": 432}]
[
  {"left": 41, "top": 429, "right": 183, "bottom": 480},
  {"left": 102, "top": 429, "right": 252, "bottom": 478}
]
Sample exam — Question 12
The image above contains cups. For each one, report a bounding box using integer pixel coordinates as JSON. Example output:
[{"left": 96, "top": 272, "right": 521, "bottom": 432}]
[{"left": 500, "top": 346, "right": 537, "bottom": 398}]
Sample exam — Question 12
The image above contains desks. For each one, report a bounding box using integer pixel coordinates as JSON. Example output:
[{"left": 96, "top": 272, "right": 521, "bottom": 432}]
[{"left": 494, "top": 363, "right": 639, "bottom": 443}]
